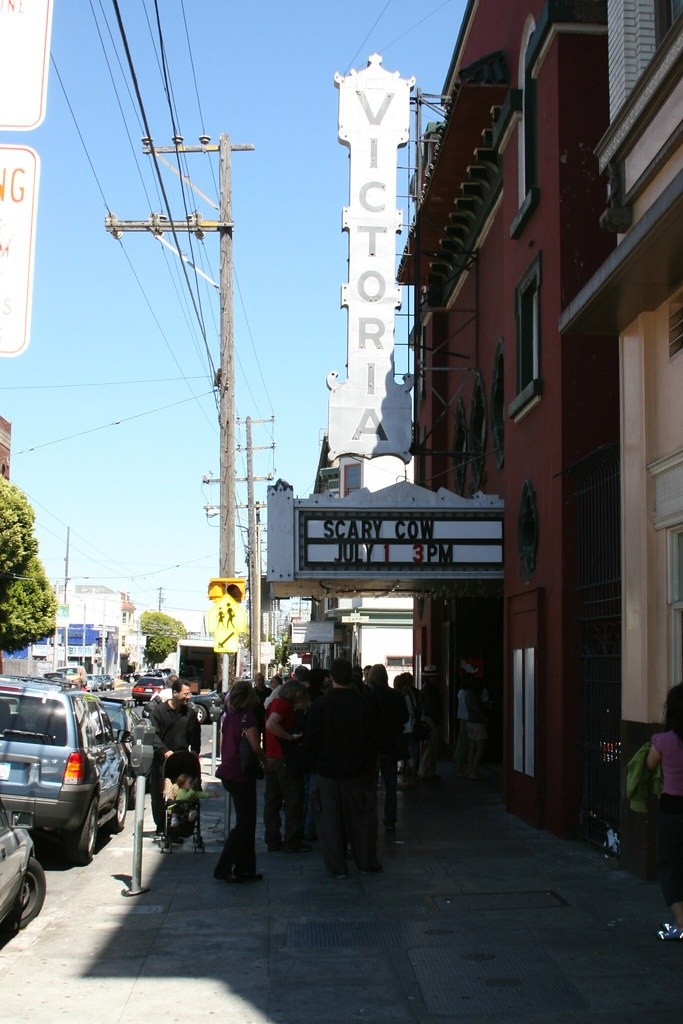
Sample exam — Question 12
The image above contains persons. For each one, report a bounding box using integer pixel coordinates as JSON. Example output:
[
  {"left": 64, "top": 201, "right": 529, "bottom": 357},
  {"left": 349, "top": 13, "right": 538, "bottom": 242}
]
[
  {"left": 255, "top": 659, "right": 439, "bottom": 881},
  {"left": 647, "top": 684, "right": 683, "bottom": 942},
  {"left": 212, "top": 682, "right": 265, "bottom": 883},
  {"left": 456, "top": 676, "right": 489, "bottom": 779},
  {"left": 151, "top": 679, "right": 202, "bottom": 834},
  {"left": 170, "top": 773, "right": 220, "bottom": 825}
]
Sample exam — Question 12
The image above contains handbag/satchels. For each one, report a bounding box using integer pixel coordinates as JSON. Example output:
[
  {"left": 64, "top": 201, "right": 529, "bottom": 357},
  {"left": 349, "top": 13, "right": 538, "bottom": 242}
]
[
  {"left": 241, "top": 710, "right": 264, "bottom": 780},
  {"left": 414, "top": 721, "right": 431, "bottom": 740},
  {"left": 279, "top": 734, "right": 301, "bottom": 768}
]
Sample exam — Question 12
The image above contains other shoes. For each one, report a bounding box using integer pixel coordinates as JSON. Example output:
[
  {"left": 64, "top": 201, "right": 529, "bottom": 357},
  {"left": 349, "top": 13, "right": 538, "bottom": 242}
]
[
  {"left": 423, "top": 773, "right": 441, "bottom": 783},
  {"left": 386, "top": 817, "right": 395, "bottom": 830},
  {"left": 188, "top": 810, "right": 197, "bottom": 822},
  {"left": 655, "top": 922, "right": 683, "bottom": 940},
  {"left": 154, "top": 830, "right": 163, "bottom": 841},
  {"left": 468, "top": 773, "right": 484, "bottom": 779},
  {"left": 457, "top": 771, "right": 469, "bottom": 778},
  {"left": 234, "top": 869, "right": 263, "bottom": 880},
  {"left": 333, "top": 869, "right": 348, "bottom": 880},
  {"left": 266, "top": 838, "right": 283, "bottom": 851},
  {"left": 286, "top": 835, "right": 313, "bottom": 851},
  {"left": 171, "top": 818, "right": 178, "bottom": 828},
  {"left": 359, "top": 864, "right": 384, "bottom": 877},
  {"left": 213, "top": 867, "right": 242, "bottom": 883}
]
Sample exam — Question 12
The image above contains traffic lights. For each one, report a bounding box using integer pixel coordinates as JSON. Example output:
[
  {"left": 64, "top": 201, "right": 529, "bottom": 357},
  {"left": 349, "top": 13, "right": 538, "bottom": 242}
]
[{"left": 209, "top": 579, "right": 247, "bottom": 602}]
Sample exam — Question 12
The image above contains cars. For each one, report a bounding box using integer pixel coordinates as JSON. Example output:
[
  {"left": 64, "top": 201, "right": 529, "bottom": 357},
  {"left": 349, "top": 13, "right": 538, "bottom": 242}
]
[
  {"left": 132, "top": 676, "right": 168, "bottom": 707},
  {"left": 102, "top": 674, "right": 115, "bottom": 690},
  {"left": 0, "top": 797, "right": 47, "bottom": 933},
  {"left": 93, "top": 674, "right": 107, "bottom": 691},
  {"left": 263, "top": 671, "right": 295, "bottom": 689},
  {"left": 187, "top": 690, "right": 228, "bottom": 725},
  {"left": 86, "top": 674, "right": 99, "bottom": 692},
  {"left": 120, "top": 668, "right": 172, "bottom": 683},
  {"left": 95, "top": 696, "right": 156, "bottom": 811}
]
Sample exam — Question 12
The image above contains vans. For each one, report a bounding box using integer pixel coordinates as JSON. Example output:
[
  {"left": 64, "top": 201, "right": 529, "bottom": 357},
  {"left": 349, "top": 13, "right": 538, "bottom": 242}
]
[{"left": 55, "top": 666, "right": 88, "bottom": 691}]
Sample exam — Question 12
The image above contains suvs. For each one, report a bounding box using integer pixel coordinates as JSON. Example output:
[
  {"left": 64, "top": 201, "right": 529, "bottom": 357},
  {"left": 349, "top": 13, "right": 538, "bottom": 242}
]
[{"left": 0, "top": 674, "right": 135, "bottom": 868}]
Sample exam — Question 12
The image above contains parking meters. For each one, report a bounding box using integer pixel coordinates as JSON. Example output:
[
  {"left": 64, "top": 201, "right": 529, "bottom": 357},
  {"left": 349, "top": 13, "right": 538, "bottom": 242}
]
[{"left": 124, "top": 718, "right": 151, "bottom": 896}]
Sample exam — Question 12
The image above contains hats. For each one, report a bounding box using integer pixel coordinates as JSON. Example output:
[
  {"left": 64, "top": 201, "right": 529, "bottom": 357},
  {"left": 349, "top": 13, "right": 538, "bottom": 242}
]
[{"left": 420, "top": 665, "right": 440, "bottom": 676}]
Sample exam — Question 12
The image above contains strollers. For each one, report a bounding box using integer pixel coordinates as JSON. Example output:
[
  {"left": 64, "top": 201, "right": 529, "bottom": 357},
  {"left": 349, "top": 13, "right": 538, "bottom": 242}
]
[{"left": 157, "top": 749, "right": 206, "bottom": 855}]
